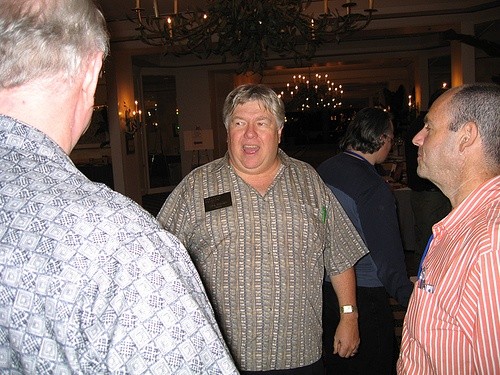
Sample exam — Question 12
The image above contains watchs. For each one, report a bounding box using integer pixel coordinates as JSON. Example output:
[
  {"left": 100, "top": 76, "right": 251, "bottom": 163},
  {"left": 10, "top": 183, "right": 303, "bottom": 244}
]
[{"left": 339, "top": 304, "right": 359, "bottom": 314}]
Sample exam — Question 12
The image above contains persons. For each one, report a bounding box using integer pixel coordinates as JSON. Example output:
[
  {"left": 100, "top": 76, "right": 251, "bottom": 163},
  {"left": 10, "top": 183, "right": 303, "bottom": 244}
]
[
  {"left": 157, "top": 84, "right": 370, "bottom": 375},
  {"left": 396, "top": 82, "right": 500, "bottom": 375},
  {"left": 0, "top": 0, "right": 242, "bottom": 375},
  {"left": 316, "top": 106, "right": 414, "bottom": 375},
  {"left": 405, "top": 88, "right": 450, "bottom": 277}
]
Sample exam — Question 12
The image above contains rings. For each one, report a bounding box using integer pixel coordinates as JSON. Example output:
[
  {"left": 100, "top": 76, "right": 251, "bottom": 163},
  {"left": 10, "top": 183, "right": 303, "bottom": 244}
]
[{"left": 352, "top": 351, "right": 357, "bottom": 354}]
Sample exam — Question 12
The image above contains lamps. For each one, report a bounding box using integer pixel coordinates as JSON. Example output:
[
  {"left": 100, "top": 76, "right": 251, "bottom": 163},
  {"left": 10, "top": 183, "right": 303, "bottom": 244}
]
[{"left": 130, "top": 0, "right": 379, "bottom": 75}]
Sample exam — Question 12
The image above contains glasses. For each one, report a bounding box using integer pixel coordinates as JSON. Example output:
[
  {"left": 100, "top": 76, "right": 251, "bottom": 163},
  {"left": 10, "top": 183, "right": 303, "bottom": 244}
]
[{"left": 383, "top": 134, "right": 397, "bottom": 146}]
